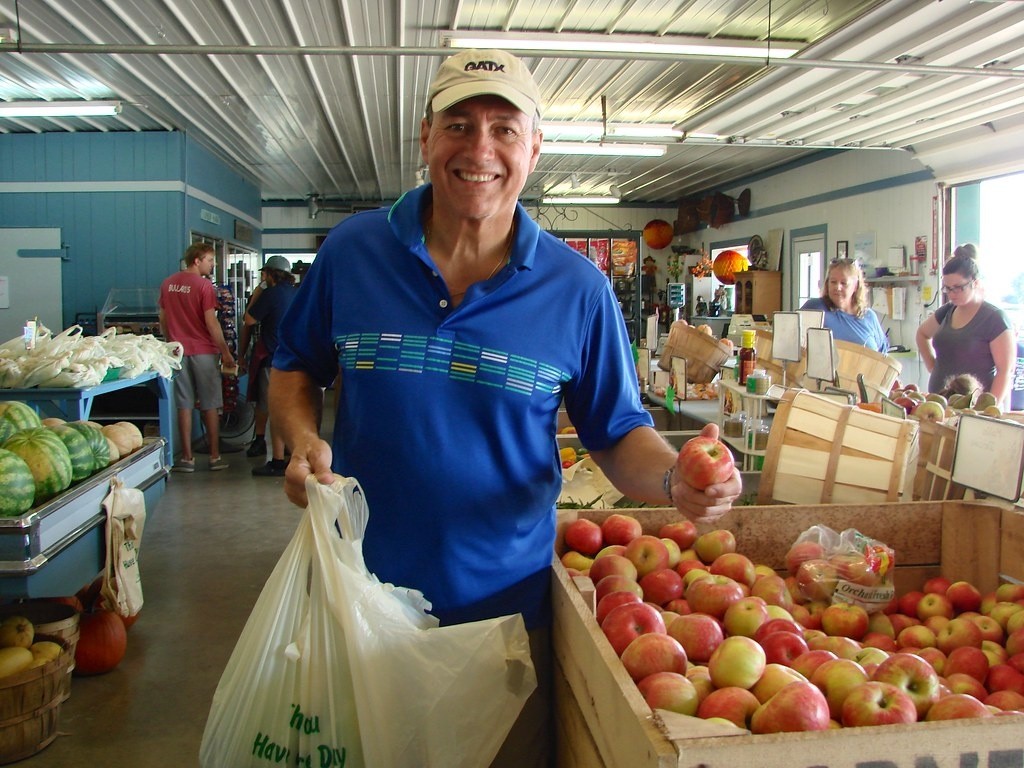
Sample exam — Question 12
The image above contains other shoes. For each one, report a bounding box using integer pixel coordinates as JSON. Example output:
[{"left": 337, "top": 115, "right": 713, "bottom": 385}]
[
  {"left": 246, "top": 439, "right": 267, "bottom": 457},
  {"left": 252, "top": 459, "right": 287, "bottom": 475}
]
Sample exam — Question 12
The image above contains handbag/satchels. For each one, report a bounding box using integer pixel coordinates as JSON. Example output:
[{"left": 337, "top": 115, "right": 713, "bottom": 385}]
[
  {"left": 0, "top": 325, "right": 184, "bottom": 387},
  {"left": 199, "top": 471, "right": 538, "bottom": 768}
]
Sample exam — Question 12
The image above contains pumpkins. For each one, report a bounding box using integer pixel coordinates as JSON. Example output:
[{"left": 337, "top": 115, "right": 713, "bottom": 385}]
[
  {"left": 0, "top": 577, "right": 138, "bottom": 680},
  {"left": 42, "top": 417, "right": 142, "bottom": 464}
]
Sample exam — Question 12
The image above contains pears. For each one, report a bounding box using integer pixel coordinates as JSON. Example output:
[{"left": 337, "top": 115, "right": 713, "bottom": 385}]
[{"left": 953, "top": 388, "right": 997, "bottom": 411}]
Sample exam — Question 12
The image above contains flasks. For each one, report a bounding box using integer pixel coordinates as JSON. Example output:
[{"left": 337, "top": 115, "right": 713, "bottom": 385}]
[{"left": 910, "top": 255, "right": 919, "bottom": 276}]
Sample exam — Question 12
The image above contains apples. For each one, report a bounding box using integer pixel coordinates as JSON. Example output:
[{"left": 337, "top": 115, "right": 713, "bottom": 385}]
[
  {"left": 676, "top": 319, "right": 732, "bottom": 350},
  {"left": 889, "top": 381, "right": 961, "bottom": 424},
  {"left": 677, "top": 435, "right": 734, "bottom": 491},
  {"left": 964, "top": 406, "right": 1001, "bottom": 418},
  {"left": 559, "top": 515, "right": 1024, "bottom": 734}
]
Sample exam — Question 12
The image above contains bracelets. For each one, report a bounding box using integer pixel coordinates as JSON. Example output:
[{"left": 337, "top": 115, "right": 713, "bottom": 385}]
[{"left": 663, "top": 466, "right": 675, "bottom": 503}]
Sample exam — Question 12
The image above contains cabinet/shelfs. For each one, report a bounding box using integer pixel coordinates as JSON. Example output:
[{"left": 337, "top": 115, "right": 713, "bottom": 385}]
[{"left": 734, "top": 270, "right": 782, "bottom": 324}]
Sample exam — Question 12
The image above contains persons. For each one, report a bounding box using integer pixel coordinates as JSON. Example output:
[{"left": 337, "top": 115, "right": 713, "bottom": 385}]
[
  {"left": 799, "top": 258, "right": 887, "bottom": 353},
  {"left": 238, "top": 256, "right": 298, "bottom": 476},
  {"left": 159, "top": 243, "right": 235, "bottom": 472},
  {"left": 266, "top": 49, "right": 742, "bottom": 768},
  {"left": 1011, "top": 342, "right": 1024, "bottom": 410},
  {"left": 916, "top": 243, "right": 1016, "bottom": 406}
]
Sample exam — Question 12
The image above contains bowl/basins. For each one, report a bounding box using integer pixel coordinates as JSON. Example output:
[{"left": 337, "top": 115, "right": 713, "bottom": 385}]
[{"left": 876, "top": 268, "right": 888, "bottom": 277}]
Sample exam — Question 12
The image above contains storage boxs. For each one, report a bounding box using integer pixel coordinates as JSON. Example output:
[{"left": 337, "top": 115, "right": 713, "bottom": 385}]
[{"left": 545, "top": 500, "right": 1024, "bottom": 768}]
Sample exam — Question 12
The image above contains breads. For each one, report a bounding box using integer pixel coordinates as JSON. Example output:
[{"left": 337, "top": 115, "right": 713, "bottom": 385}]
[{"left": 655, "top": 383, "right": 718, "bottom": 401}]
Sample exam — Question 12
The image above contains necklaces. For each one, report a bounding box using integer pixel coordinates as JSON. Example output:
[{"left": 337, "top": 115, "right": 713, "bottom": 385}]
[{"left": 452, "top": 222, "right": 514, "bottom": 297}]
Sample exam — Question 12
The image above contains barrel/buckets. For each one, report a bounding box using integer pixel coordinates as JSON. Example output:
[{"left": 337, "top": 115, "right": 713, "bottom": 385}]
[
  {"left": 657, "top": 324, "right": 732, "bottom": 386},
  {"left": 32, "top": 601, "right": 80, "bottom": 702},
  {"left": 904, "top": 411, "right": 986, "bottom": 501},
  {"left": 759, "top": 385, "right": 916, "bottom": 509},
  {"left": 796, "top": 341, "right": 901, "bottom": 409},
  {"left": 0, "top": 634, "right": 74, "bottom": 763}
]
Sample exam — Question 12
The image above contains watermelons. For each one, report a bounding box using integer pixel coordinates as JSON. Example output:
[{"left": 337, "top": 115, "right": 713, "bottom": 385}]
[{"left": 0, "top": 400, "right": 111, "bottom": 517}]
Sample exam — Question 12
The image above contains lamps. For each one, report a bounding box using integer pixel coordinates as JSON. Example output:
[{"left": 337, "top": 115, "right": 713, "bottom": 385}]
[
  {"left": 540, "top": 142, "right": 667, "bottom": 158},
  {"left": 540, "top": 196, "right": 621, "bottom": 204},
  {"left": 0, "top": 100, "right": 122, "bottom": 117},
  {"left": 439, "top": 29, "right": 804, "bottom": 60},
  {"left": 610, "top": 178, "right": 622, "bottom": 198},
  {"left": 414, "top": 171, "right": 425, "bottom": 188},
  {"left": 537, "top": 120, "right": 672, "bottom": 136},
  {"left": 570, "top": 173, "right": 581, "bottom": 189}
]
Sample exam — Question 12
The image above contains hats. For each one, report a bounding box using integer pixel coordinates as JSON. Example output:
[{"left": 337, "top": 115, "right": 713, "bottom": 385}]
[
  {"left": 258, "top": 255, "right": 292, "bottom": 275},
  {"left": 425, "top": 47, "right": 544, "bottom": 123}
]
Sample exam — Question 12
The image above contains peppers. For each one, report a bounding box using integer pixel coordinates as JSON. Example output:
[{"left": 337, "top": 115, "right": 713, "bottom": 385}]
[{"left": 554, "top": 424, "right": 586, "bottom": 468}]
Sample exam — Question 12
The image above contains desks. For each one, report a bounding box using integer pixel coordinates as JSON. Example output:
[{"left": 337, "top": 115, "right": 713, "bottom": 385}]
[
  {"left": 0, "top": 369, "right": 174, "bottom": 467},
  {"left": 0, "top": 436, "right": 171, "bottom": 600}
]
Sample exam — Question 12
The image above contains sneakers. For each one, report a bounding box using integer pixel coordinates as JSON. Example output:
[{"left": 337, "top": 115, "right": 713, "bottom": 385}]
[
  {"left": 208, "top": 455, "right": 230, "bottom": 470},
  {"left": 171, "top": 457, "right": 195, "bottom": 472}
]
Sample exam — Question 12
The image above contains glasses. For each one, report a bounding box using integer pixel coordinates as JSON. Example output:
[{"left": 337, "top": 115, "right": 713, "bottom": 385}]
[
  {"left": 942, "top": 277, "right": 976, "bottom": 294},
  {"left": 830, "top": 257, "right": 859, "bottom": 267}
]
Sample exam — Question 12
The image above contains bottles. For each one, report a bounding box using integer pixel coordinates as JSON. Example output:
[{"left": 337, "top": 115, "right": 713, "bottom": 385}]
[{"left": 739, "top": 331, "right": 756, "bottom": 385}]
